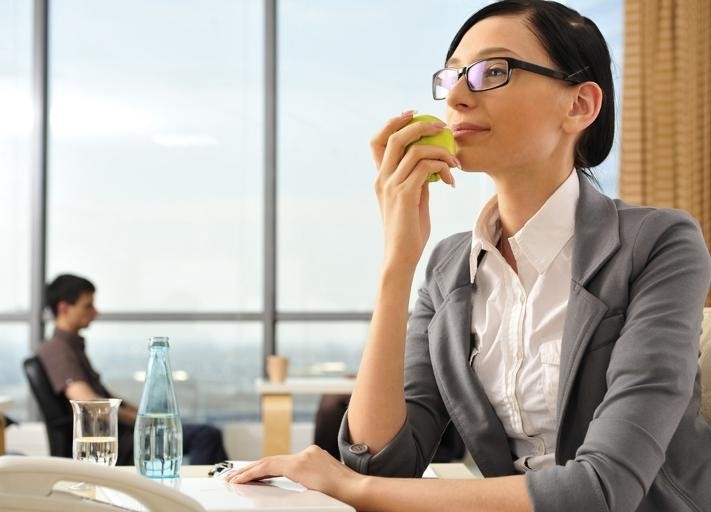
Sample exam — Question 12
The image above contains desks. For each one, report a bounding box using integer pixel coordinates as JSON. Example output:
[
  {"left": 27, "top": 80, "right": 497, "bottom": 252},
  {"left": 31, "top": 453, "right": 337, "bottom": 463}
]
[
  {"left": 256, "top": 376, "right": 355, "bottom": 459},
  {"left": 1, "top": 456, "right": 484, "bottom": 512}
]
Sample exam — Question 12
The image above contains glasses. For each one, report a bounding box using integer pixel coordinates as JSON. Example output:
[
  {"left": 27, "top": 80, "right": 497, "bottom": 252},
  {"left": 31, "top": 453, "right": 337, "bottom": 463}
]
[{"left": 431, "top": 56, "right": 583, "bottom": 101}]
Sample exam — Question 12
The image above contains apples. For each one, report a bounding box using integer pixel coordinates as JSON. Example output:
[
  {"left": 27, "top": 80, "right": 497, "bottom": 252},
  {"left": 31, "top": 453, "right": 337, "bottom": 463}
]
[{"left": 394, "top": 115, "right": 457, "bottom": 182}]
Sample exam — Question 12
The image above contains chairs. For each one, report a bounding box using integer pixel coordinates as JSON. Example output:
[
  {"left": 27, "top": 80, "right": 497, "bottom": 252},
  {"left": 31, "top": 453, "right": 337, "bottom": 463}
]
[{"left": 22, "top": 345, "right": 134, "bottom": 466}]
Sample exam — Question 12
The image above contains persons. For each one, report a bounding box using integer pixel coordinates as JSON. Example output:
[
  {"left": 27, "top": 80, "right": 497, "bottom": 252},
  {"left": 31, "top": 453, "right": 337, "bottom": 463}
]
[
  {"left": 39, "top": 272, "right": 228, "bottom": 468},
  {"left": 227, "top": 1, "right": 711, "bottom": 512}
]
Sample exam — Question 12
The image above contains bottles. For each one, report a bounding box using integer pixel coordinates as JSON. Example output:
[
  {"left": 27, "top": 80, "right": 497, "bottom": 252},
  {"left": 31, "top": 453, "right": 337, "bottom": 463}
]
[{"left": 132, "top": 334, "right": 185, "bottom": 479}]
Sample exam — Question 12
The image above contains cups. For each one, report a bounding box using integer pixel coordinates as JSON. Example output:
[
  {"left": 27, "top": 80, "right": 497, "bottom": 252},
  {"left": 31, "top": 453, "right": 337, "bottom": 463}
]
[{"left": 67, "top": 396, "right": 123, "bottom": 470}]
